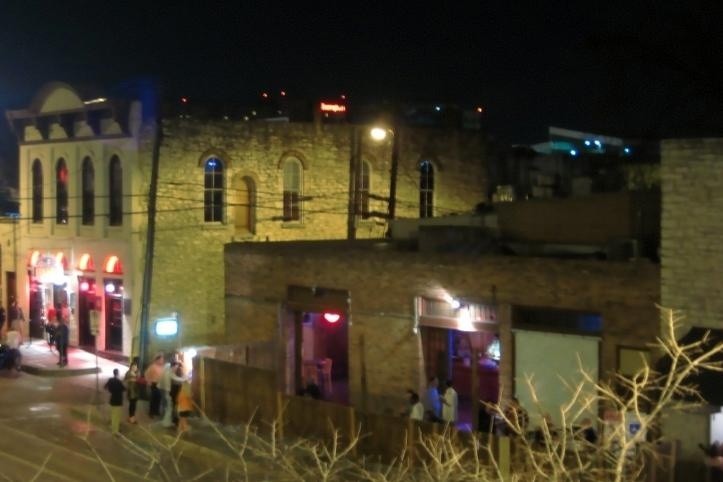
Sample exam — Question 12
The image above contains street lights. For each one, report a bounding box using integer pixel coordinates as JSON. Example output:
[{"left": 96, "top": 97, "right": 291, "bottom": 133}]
[{"left": 369, "top": 118, "right": 398, "bottom": 220}]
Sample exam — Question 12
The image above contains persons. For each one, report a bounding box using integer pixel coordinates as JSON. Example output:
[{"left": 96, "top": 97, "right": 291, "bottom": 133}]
[
  {"left": 103, "top": 350, "right": 193, "bottom": 437},
  {"left": 439, "top": 380, "right": 458, "bottom": 426},
  {"left": 478, "top": 397, "right": 559, "bottom": 452},
  {"left": 297, "top": 377, "right": 321, "bottom": 400},
  {"left": 409, "top": 394, "right": 424, "bottom": 420},
  {"left": 0, "top": 296, "right": 26, "bottom": 374},
  {"left": 46, "top": 301, "right": 71, "bottom": 367}
]
